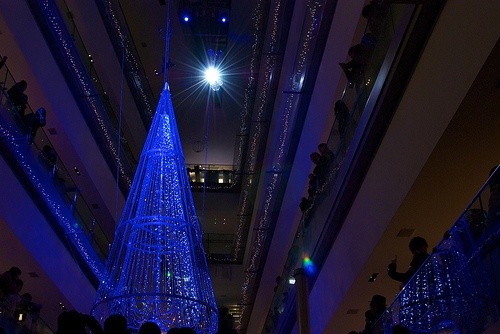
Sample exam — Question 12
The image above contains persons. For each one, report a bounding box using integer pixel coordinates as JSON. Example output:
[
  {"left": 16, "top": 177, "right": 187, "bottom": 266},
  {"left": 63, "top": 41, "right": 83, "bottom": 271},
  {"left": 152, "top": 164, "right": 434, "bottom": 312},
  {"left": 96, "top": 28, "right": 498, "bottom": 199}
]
[{"left": 1, "top": 0, "right": 500, "bottom": 334}]
